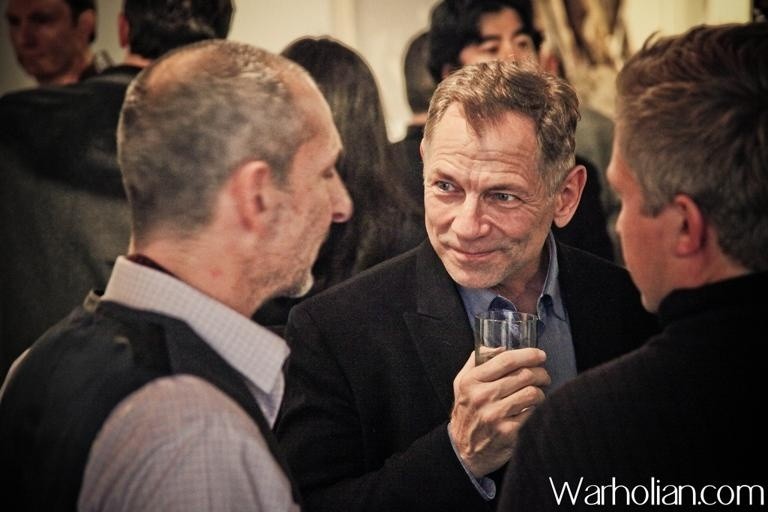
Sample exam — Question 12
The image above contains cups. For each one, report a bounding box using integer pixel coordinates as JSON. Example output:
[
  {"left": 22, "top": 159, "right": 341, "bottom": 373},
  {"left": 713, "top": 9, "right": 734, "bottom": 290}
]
[{"left": 473, "top": 308, "right": 540, "bottom": 364}]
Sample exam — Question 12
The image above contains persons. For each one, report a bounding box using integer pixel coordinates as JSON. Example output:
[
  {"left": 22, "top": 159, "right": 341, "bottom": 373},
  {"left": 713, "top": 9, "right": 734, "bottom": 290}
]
[
  {"left": 0, "top": 1, "right": 768, "bottom": 378},
  {"left": 271, "top": 60, "right": 661, "bottom": 512},
  {"left": 0, "top": 39, "right": 351, "bottom": 511},
  {"left": 499, "top": 20, "right": 767, "bottom": 512}
]
